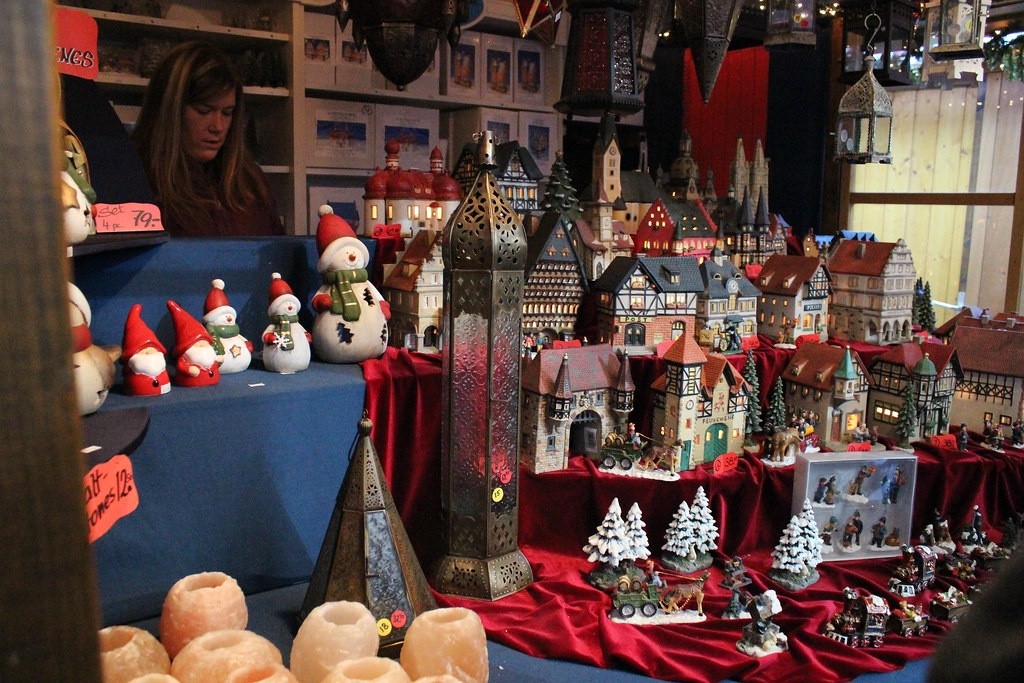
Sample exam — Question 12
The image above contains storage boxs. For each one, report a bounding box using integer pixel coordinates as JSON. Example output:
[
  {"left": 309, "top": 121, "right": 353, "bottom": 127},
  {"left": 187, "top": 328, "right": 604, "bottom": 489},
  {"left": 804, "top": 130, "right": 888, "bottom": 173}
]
[{"left": 793, "top": 450, "right": 917, "bottom": 565}]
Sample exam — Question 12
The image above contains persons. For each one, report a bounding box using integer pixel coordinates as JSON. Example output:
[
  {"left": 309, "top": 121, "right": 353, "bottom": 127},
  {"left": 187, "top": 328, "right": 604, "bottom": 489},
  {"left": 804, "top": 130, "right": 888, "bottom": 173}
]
[
  {"left": 130, "top": 39, "right": 288, "bottom": 236},
  {"left": 851, "top": 419, "right": 878, "bottom": 445},
  {"left": 644, "top": 559, "right": 663, "bottom": 589},
  {"left": 957, "top": 422, "right": 969, "bottom": 451},
  {"left": 880, "top": 464, "right": 904, "bottom": 504},
  {"left": 983, "top": 419, "right": 1004, "bottom": 450},
  {"left": 813, "top": 477, "right": 825, "bottom": 504},
  {"left": 972, "top": 504, "right": 983, "bottom": 546},
  {"left": 1011, "top": 420, "right": 1024, "bottom": 445},
  {"left": 842, "top": 517, "right": 858, "bottom": 543},
  {"left": 825, "top": 476, "right": 840, "bottom": 506},
  {"left": 627, "top": 422, "right": 643, "bottom": 449},
  {"left": 820, "top": 516, "right": 838, "bottom": 546},
  {"left": 792, "top": 426, "right": 808, "bottom": 453},
  {"left": 720, "top": 320, "right": 742, "bottom": 351},
  {"left": 870, "top": 517, "right": 887, "bottom": 548},
  {"left": 933, "top": 507, "right": 944, "bottom": 546},
  {"left": 788, "top": 407, "right": 817, "bottom": 435},
  {"left": 848, "top": 511, "right": 863, "bottom": 546},
  {"left": 761, "top": 428, "right": 776, "bottom": 461},
  {"left": 852, "top": 465, "right": 872, "bottom": 495}
]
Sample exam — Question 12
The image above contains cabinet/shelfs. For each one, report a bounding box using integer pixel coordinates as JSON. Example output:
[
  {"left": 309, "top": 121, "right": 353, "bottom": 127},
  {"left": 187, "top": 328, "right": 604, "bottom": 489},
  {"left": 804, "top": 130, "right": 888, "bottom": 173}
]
[{"left": 50, "top": 1, "right": 441, "bottom": 630}]
